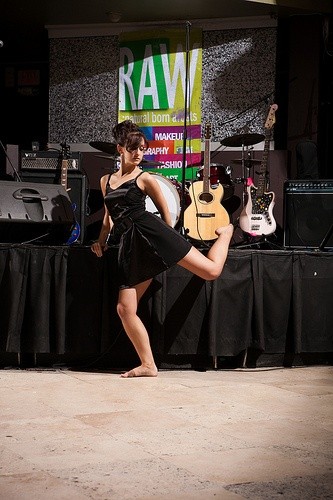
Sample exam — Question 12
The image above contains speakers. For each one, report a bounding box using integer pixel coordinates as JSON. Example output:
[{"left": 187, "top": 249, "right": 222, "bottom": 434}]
[
  {"left": 0, "top": 172, "right": 87, "bottom": 245},
  {"left": 282, "top": 180, "right": 333, "bottom": 251}
]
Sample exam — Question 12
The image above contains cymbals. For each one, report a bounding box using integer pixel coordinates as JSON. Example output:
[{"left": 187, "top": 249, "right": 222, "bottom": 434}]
[
  {"left": 89, "top": 141, "right": 120, "bottom": 156},
  {"left": 220, "top": 133, "right": 265, "bottom": 147},
  {"left": 230, "top": 157, "right": 262, "bottom": 165}
]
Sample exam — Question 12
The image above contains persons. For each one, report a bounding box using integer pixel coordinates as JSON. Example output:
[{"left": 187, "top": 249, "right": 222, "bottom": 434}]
[{"left": 91, "top": 120, "right": 234, "bottom": 378}]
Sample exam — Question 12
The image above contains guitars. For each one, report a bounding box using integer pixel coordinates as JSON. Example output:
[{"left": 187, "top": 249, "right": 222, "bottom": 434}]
[
  {"left": 184, "top": 122, "right": 230, "bottom": 241},
  {"left": 239, "top": 104, "right": 277, "bottom": 236},
  {"left": 60, "top": 138, "right": 81, "bottom": 245}
]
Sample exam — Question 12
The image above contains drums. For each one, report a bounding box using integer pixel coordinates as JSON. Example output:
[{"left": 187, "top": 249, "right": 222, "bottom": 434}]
[
  {"left": 195, "top": 162, "right": 234, "bottom": 201},
  {"left": 145, "top": 172, "right": 192, "bottom": 231}
]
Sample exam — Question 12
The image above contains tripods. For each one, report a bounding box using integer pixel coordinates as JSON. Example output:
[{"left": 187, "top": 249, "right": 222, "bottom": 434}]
[{"left": 235, "top": 166, "right": 283, "bottom": 249}]
